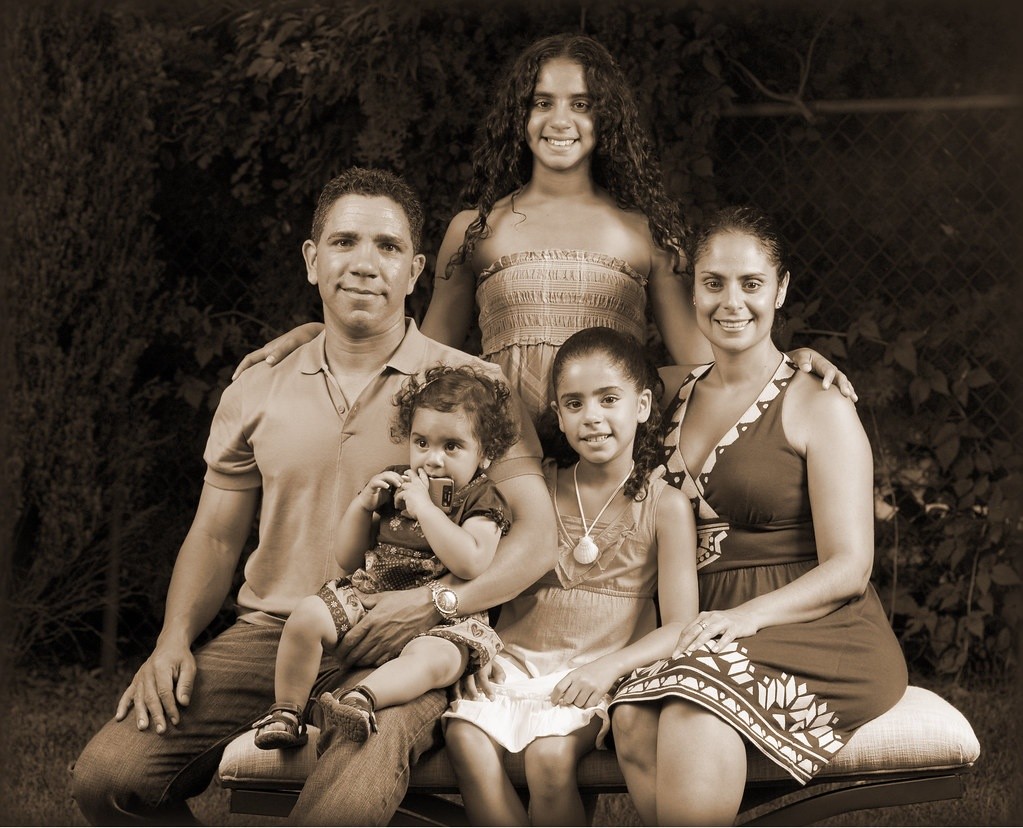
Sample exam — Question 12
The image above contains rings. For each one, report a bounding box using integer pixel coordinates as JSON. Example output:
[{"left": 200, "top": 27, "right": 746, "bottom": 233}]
[{"left": 697, "top": 621, "right": 707, "bottom": 630}]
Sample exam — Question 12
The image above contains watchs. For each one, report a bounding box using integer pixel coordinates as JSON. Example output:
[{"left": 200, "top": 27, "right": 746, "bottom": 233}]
[{"left": 422, "top": 580, "right": 458, "bottom": 624}]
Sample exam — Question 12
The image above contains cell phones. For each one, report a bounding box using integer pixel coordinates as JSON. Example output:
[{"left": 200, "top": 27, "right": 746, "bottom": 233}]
[{"left": 392, "top": 475, "right": 454, "bottom": 514}]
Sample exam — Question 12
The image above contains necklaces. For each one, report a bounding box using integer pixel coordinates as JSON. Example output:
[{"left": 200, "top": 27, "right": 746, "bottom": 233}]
[{"left": 573, "top": 459, "right": 635, "bottom": 564}]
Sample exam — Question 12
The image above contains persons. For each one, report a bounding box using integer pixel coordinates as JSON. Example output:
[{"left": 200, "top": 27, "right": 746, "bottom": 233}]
[
  {"left": 251, "top": 360, "right": 525, "bottom": 750},
  {"left": 232, "top": 35, "right": 859, "bottom": 422},
  {"left": 65, "top": 166, "right": 559, "bottom": 827},
  {"left": 436, "top": 327, "right": 699, "bottom": 828},
  {"left": 607, "top": 207, "right": 908, "bottom": 827}
]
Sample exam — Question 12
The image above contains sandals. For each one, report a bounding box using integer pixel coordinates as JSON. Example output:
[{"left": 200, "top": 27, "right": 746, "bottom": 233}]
[
  {"left": 251, "top": 702, "right": 308, "bottom": 750},
  {"left": 321, "top": 685, "right": 379, "bottom": 743}
]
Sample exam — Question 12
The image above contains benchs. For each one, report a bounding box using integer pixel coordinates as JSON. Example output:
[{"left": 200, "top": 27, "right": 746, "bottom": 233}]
[{"left": 219, "top": 685, "right": 981, "bottom": 827}]
